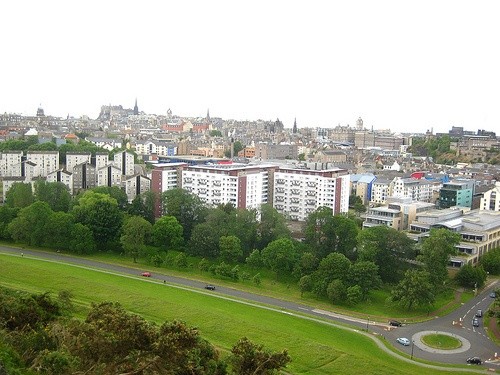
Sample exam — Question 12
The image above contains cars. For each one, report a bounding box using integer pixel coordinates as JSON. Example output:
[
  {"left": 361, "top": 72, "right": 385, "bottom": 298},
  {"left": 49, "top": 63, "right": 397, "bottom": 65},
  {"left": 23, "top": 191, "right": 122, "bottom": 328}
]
[
  {"left": 389, "top": 319, "right": 401, "bottom": 327},
  {"left": 396, "top": 338, "right": 411, "bottom": 346},
  {"left": 207, "top": 284, "right": 215, "bottom": 289},
  {"left": 466, "top": 356, "right": 482, "bottom": 365},
  {"left": 141, "top": 272, "right": 151, "bottom": 277},
  {"left": 476, "top": 309, "right": 482, "bottom": 317},
  {"left": 473, "top": 317, "right": 479, "bottom": 326}
]
